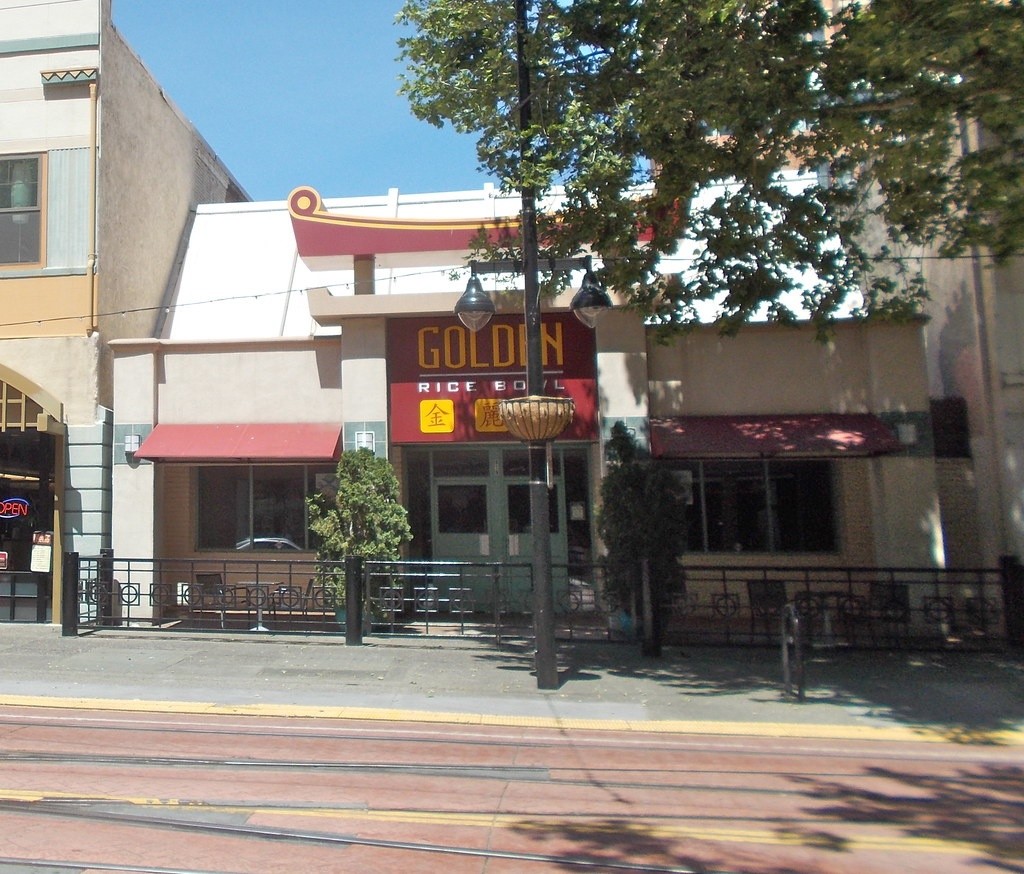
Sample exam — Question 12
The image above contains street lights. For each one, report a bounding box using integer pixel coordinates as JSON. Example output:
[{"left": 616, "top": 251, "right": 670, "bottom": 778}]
[{"left": 455, "top": 252, "right": 614, "bottom": 689}]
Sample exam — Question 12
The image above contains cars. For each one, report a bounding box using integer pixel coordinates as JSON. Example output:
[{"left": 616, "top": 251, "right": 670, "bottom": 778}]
[{"left": 235, "top": 538, "right": 303, "bottom": 552}]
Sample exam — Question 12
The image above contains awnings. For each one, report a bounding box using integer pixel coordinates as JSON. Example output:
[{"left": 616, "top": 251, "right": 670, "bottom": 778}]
[
  {"left": 133, "top": 422, "right": 344, "bottom": 462},
  {"left": 647, "top": 414, "right": 905, "bottom": 459}
]
